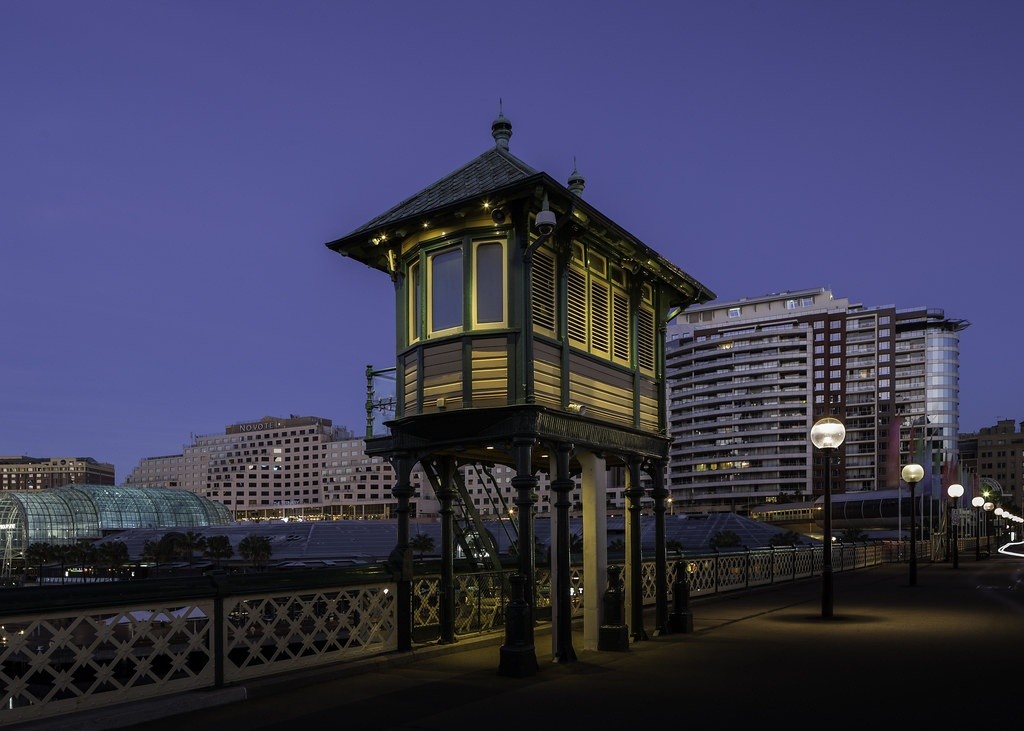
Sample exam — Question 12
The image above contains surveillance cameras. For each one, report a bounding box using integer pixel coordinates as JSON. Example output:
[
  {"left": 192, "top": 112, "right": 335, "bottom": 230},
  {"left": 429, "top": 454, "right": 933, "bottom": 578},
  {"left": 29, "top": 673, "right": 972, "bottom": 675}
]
[{"left": 535, "top": 210, "right": 557, "bottom": 235}]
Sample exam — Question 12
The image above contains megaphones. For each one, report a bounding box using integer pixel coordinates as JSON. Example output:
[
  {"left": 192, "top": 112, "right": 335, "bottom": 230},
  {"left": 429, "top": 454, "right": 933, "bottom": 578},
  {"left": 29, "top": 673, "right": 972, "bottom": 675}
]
[
  {"left": 568, "top": 403, "right": 586, "bottom": 415},
  {"left": 491, "top": 206, "right": 509, "bottom": 224}
]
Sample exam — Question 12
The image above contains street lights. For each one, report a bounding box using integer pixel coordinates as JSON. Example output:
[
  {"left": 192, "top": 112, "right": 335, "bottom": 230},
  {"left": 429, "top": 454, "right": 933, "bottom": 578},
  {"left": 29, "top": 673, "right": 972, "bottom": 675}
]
[
  {"left": 810, "top": 417, "right": 847, "bottom": 620},
  {"left": 901, "top": 463, "right": 924, "bottom": 588},
  {"left": 947, "top": 483, "right": 965, "bottom": 570},
  {"left": 509, "top": 509, "right": 513, "bottom": 516},
  {"left": 971, "top": 496, "right": 985, "bottom": 561},
  {"left": 668, "top": 498, "right": 673, "bottom": 516},
  {"left": 1002, "top": 511, "right": 1024, "bottom": 544},
  {"left": 993, "top": 507, "right": 1004, "bottom": 550},
  {"left": 983, "top": 502, "right": 995, "bottom": 554}
]
